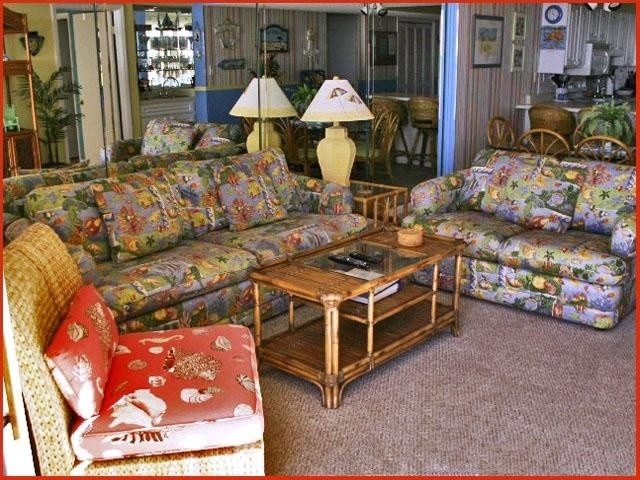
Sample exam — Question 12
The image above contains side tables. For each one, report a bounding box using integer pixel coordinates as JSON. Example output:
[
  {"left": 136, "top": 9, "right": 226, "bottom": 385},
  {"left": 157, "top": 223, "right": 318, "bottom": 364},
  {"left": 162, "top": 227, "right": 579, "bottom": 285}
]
[{"left": 348, "top": 180, "right": 409, "bottom": 229}]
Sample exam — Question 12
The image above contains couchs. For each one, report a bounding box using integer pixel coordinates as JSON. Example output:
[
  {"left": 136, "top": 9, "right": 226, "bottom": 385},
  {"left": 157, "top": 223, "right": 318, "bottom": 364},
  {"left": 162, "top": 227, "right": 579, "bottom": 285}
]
[
  {"left": 106, "top": 117, "right": 248, "bottom": 162},
  {"left": 3, "top": 223, "right": 266, "bottom": 478},
  {"left": 3, "top": 153, "right": 196, "bottom": 228},
  {"left": 401, "top": 147, "right": 636, "bottom": 330},
  {"left": 5, "top": 148, "right": 368, "bottom": 334}
]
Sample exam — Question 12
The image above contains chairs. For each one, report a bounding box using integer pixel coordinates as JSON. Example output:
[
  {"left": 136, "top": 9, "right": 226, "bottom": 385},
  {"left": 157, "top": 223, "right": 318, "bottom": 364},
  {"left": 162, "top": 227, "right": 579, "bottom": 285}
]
[
  {"left": 243, "top": 95, "right": 438, "bottom": 183},
  {"left": 486, "top": 103, "right": 636, "bottom": 165}
]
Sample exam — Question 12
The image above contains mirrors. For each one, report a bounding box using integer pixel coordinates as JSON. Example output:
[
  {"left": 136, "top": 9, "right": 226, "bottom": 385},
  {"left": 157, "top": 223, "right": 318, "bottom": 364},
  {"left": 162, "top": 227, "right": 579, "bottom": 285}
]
[{"left": 2, "top": 4, "right": 441, "bottom": 209}]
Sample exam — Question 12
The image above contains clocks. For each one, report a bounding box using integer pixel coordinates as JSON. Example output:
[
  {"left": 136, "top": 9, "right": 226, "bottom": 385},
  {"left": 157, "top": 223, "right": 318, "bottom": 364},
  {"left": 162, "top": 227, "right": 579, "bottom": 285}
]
[{"left": 545, "top": 5, "right": 563, "bottom": 24}]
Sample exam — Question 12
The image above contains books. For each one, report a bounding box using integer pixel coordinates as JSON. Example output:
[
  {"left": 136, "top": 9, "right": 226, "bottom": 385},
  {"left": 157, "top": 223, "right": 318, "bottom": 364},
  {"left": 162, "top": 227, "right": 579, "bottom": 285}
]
[{"left": 348, "top": 278, "right": 401, "bottom": 306}]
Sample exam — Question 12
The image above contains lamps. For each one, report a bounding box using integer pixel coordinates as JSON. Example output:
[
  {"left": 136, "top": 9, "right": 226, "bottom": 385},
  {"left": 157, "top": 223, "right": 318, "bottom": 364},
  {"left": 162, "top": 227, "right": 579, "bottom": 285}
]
[
  {"left": 300, "top": 76, "right": 376, "bottom": 187},
  {"left": 229, "top": 75, "right": 298, "bottom": 154}
]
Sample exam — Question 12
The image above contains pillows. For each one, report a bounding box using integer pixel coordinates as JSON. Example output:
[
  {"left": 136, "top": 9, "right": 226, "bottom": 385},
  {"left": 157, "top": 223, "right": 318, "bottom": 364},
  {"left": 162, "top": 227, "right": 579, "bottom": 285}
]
[
  {"left": 143, "top": 125, "right": 240, "bottom": 156},
  {"left": 209, "top": 158, "right": 289, "bottom": 231},
  {"left": 480, "top": 155, "right": 588, "bottom": 235},
  {"left": 90, "top": 174, "right": 185, "bottom": 268}
]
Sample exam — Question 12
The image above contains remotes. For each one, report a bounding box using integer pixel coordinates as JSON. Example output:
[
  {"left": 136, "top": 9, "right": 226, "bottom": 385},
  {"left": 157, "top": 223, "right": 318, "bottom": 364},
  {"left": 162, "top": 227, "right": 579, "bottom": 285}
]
[
  {"left": 349, "top": 250, "right": 378, "bottom": 264},
  {"left": 330, "top": 254, "right": 372, "bottom": 270}
]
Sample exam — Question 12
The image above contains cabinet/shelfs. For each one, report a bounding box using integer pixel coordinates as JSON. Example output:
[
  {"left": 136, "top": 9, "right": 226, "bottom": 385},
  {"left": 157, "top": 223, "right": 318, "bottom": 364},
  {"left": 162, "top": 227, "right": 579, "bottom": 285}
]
[
  {"left": 1, "top": 6, "right": 42, "bottom": 178},
  {"left": 124, "top": 3, "right": 195, "bottom": 87},
  {"left": 541, "top": 3, "right": 636, "bottom": 67}
]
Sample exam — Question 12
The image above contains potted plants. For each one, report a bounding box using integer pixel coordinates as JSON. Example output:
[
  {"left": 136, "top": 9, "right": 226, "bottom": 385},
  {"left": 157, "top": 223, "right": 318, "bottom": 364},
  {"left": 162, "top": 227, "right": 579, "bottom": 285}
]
[{"left": 12, "top": 65, "right": 86, "bottom": 169}]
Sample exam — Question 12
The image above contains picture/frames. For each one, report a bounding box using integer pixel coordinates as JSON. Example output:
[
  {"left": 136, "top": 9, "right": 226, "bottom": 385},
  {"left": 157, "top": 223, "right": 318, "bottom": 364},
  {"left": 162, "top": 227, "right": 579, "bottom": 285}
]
[{"left": 472, "top": 12, "right": 527, "bottom": 72}]
